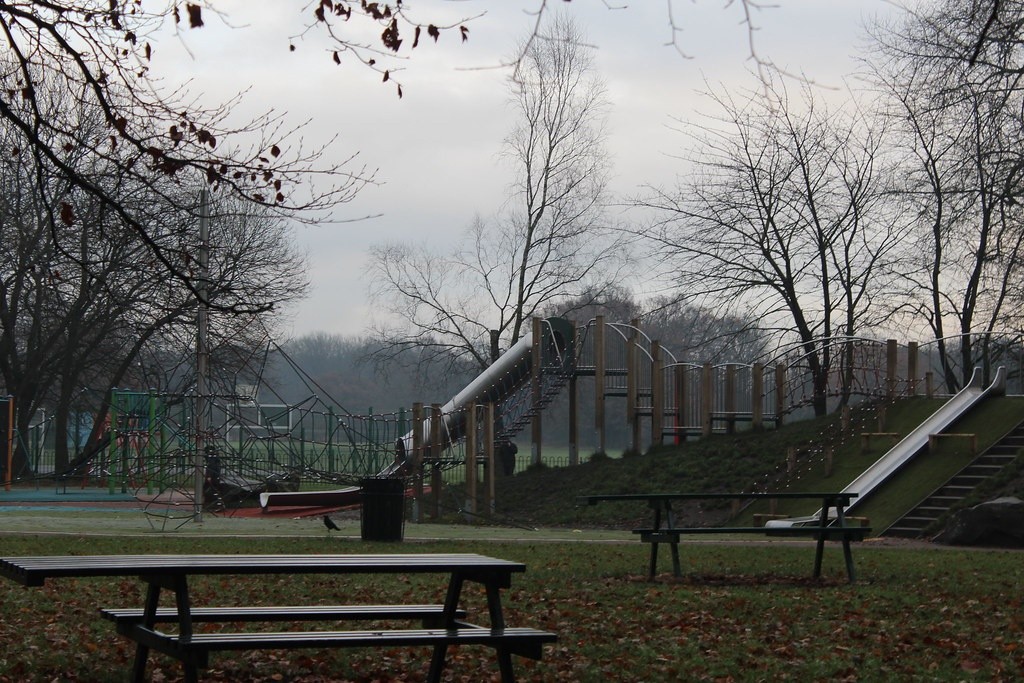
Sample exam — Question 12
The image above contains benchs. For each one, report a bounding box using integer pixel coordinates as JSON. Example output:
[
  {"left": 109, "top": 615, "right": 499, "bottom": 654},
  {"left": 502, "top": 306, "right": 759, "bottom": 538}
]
[
  {"left": 633, "top": 524, "right": 874, "bottom": 585},
  {"left": 100, "top": 603, "right": 466, "bottom": 677},
  {"left": 158, "top": 626, "right": 560, "bottom": 683}
]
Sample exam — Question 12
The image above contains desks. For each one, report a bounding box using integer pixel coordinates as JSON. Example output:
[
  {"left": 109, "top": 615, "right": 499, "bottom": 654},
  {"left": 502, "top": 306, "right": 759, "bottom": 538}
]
[
  {"left": 0, "top": 543, "right": 528, "bottom": 683},
  {"left": 576, "top": 491, "right": 860, "bottom": 529}
]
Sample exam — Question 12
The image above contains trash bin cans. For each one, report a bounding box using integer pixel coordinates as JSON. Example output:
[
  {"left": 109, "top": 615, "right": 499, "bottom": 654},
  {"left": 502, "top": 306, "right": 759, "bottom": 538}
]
[{"left": 360, "top": 478, "right": 406, "bottom": 542}]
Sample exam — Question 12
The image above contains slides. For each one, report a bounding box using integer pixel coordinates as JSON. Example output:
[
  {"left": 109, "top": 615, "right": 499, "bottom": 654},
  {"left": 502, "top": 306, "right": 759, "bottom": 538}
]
[
  {"left": 257, "top": 316, "right": 578, "bottom": 508},
  {"left": 765, "top": 366, "right": 1008, "bottom": 527}
]
